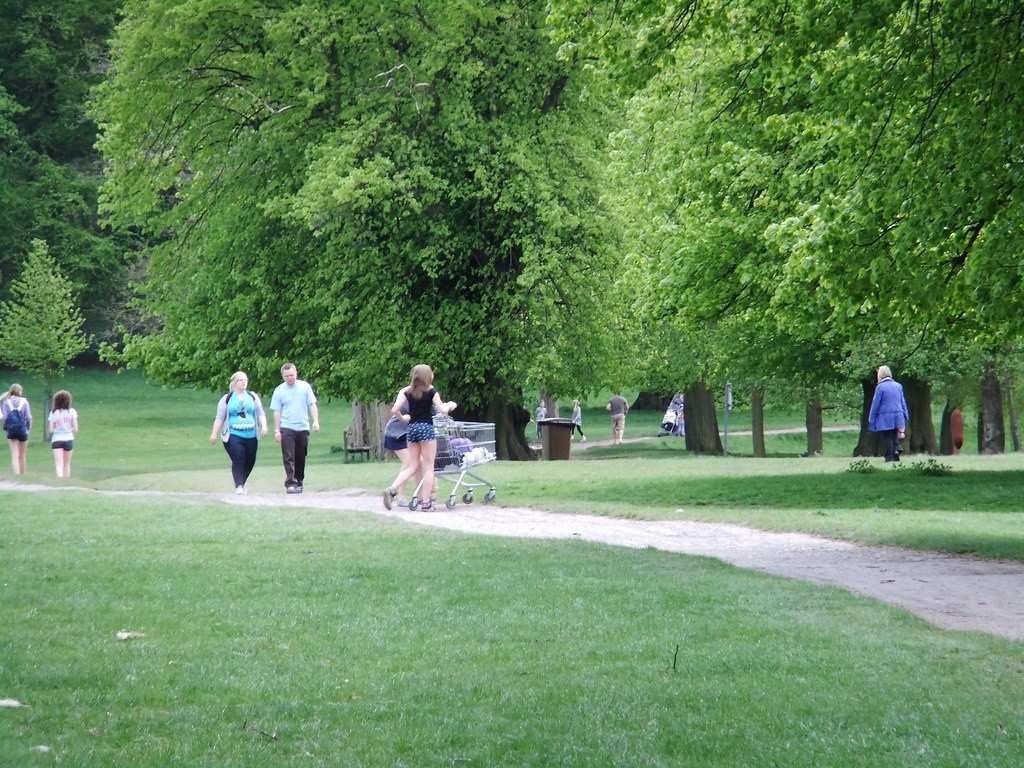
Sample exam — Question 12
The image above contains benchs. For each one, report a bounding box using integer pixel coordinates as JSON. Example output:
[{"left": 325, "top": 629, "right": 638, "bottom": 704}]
[{"left": 344, "top": 426, "right": 370, "bottom": 463}]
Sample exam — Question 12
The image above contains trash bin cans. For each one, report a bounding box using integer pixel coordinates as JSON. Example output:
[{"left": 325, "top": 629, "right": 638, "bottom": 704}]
[{"left": 536, "top": 418, "right": 576, "bottom": 460}]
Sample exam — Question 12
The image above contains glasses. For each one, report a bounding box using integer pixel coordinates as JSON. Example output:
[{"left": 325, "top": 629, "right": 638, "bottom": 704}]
[{"left": 235, "top": 378, "right": 250, "bottom": 384}]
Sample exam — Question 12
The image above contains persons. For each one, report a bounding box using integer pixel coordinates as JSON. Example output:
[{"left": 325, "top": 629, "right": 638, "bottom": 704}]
[
  {"left": 950, "top": 408, "right": 963, "bottom": 456},
  {"left": 535, "top": 400, "right": 547, "bottom": 439},
  {"left": 676, "top": 404, "right": 685, "bottom": 436},
  {"left": 606, "top": 390, "right": 628, "bottom": 446},
  {"left": 868, "top": 366, "right": 909, "bottom": 463},
  {"left": 0, "top": 384, "right": 32, "bottom": 474},
  {"left": 570, "top": 400, "right": 586, "bottom": 443},
  {"left": 383, "top": 364, "right": 457, "bottom": 512},
  {"left": 269, "top": 363, "right": 320, "bottom": 494},
  {"left": 210, "top": 371, "right": 268, "bottom": 495},
  {"left": 47, "top": 390, "right": 78, "bottom": 478}
]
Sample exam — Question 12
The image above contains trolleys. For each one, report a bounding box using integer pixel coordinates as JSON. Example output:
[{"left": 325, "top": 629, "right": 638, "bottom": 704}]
[{"left": 409, "top": 417, "right": 497, "bottom": 511}]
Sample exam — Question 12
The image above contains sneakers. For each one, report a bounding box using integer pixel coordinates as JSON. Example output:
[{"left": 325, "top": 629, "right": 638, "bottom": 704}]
[
  {"left": 422, "top": 501, "right": 439, "bottom": 512},
  {"left": 382, "top": 486, "right": 397, "bottom": 510}
]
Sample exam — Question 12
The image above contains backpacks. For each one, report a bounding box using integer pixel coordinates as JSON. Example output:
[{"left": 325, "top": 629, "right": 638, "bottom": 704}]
[{"left": 7, "top": 398, "right": 28, "bottom": 441}]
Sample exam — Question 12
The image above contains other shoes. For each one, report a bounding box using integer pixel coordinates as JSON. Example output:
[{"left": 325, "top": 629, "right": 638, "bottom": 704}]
[
  {"left": 571, "top": 434, "right": 574, "bottom": 440},
  {"left": 243, "top": 485, "right": 248, "bottom": 495},
  {"left": 236, "top": 485, "right": 243, "bottom": 494},
  {"left": 536, "top": 435, "right": 540, "bottom": 439},
  {"left": 297, "top": 486, "right": 303, "bottom": 493},
  {"left": 287, "top": 487, "right": 296, "bottom": 493},
  {"left": 398, "top": 498, "right": 409, "bottom": 507},
  {"left": 580, "top": 436, "right": 588, "bottom": 442}
]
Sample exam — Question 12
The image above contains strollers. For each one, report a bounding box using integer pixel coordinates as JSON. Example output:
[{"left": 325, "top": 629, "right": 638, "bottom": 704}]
[{"left": 657, "top": 391, "right": 685, "bottom": 436}]
